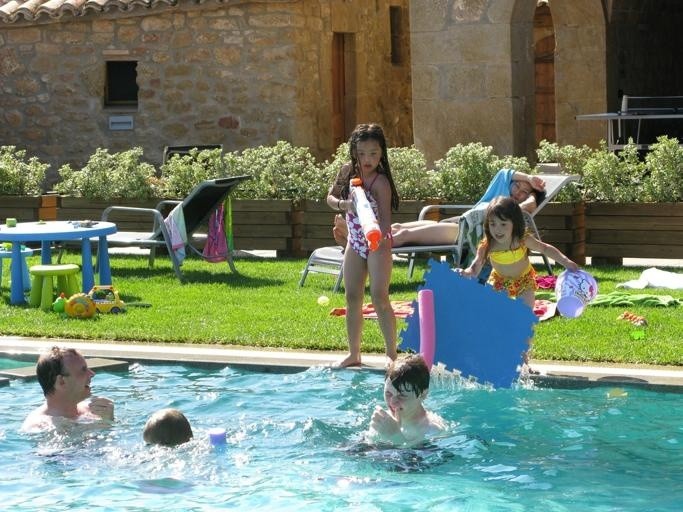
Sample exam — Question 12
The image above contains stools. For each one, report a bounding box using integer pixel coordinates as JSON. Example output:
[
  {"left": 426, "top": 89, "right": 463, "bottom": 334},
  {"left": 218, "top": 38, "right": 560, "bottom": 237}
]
[
  {"left": 0, "top": 244, "right": 33, "bottom": 295},
  {"left": 30, "top": 264, "right": 80, "bottom": 309}
]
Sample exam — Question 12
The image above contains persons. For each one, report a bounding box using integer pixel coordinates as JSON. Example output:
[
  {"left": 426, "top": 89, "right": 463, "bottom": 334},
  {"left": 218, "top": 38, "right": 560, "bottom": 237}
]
[
  {"left": 141, "top": 406, "right": 192, "bottom": 447},
  {"left": 20, "top": 345, "right": 115, "bottom": 431},
  {"left": 331, "top": 167, "right": 547, "bottom": 249},
  {"left": 325, "top": 122, "right": 401, "bottom": 371},
  {"left": 452, "top": 195, "right": 582, "bottom": 371},
  {"left": 368, "top": 355, "right": 448, "bottom": 448}
]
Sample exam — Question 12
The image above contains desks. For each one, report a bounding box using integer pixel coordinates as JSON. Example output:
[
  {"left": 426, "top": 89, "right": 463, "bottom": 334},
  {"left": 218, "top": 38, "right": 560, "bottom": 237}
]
[
  {"left": 0, "top": 221, "right": 117, "bottom": 295},
  {"left": 574, "top": 113, "right": 683, "bottom": 155}
]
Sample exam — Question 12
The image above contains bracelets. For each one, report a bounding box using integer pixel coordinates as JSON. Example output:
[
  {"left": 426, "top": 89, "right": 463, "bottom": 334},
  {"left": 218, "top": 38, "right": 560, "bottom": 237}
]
[{"left": 336, "top": 198, "right": 342, "bottom": 211}]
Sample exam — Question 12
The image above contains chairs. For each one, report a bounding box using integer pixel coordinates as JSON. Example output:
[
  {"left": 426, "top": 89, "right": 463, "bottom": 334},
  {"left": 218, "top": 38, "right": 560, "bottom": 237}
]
[
  {"left": 615, "top": 94, "right": 682, "bottom": 160},
  {"left": 161, "top": 144, "right": 223, "bottom": 175},
  {"left": 57, "top": 175, "right": 251, "bottom": 286},
  {"left": 299, "top": 174, "right": 581, "bottom": 293}
]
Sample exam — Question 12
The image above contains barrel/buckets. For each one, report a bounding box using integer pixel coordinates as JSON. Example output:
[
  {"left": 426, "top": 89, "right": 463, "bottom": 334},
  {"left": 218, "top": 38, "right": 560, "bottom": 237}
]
[{"left": 555, "top": 269, "right": 597, "bottom": 318}]
